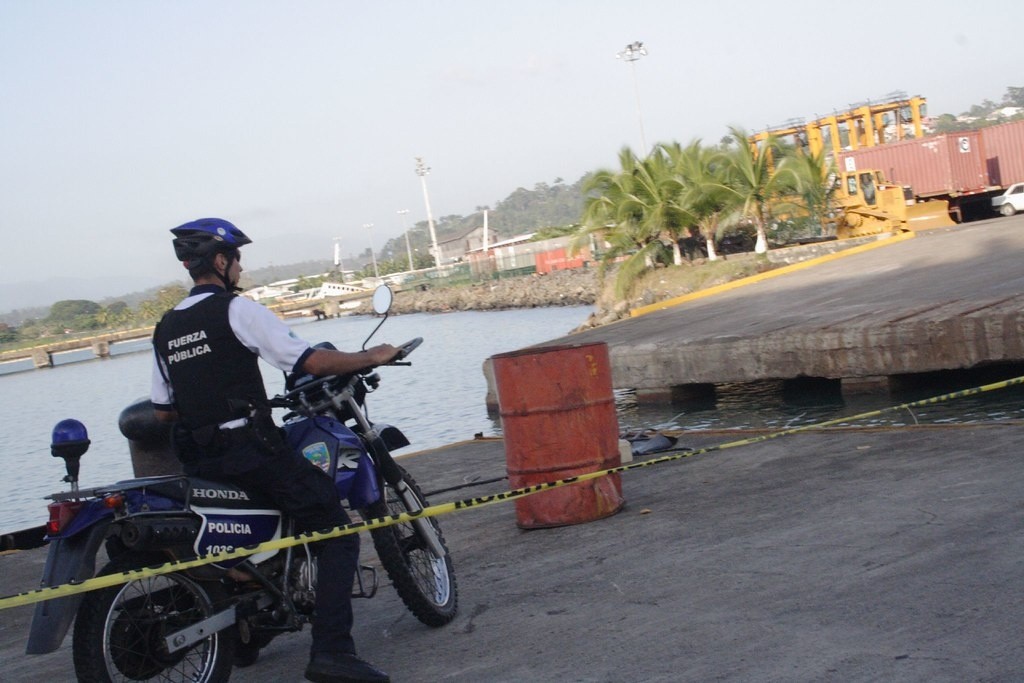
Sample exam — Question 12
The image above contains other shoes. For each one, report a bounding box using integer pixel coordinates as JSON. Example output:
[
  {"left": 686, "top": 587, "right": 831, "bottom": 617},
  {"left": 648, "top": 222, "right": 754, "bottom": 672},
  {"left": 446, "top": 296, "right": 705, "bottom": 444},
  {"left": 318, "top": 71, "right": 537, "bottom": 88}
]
[{"left": 304, "top": 652, "right": 390, "bottom": 683}]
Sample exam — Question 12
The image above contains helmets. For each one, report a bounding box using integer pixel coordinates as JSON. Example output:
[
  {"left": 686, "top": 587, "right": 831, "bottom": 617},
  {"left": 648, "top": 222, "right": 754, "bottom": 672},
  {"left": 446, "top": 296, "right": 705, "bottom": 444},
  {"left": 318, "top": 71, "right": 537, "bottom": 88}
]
[{"left": 169, "top": 217, "right": 251, "bottom": 261}]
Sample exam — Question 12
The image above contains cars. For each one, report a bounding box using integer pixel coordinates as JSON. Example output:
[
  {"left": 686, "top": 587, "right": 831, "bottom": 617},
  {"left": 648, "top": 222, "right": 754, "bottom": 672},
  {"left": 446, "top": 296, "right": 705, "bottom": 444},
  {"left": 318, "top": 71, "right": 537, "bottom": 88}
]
[{"left": 992, "top": 182, "right": 1024, "bottom": 215}]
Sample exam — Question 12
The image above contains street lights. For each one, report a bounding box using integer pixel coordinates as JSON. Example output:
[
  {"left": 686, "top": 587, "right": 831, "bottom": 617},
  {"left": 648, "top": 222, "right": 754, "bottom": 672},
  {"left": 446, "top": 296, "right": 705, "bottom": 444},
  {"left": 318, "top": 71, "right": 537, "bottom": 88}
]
[
  {"left": 397, "top": 209, "right": 414, "bottom": 271},
  {"left": 413, "top": 155, "right": 439, "bottom": 266},
  {"left": 615, "top": 40, "right": 648, "bottom": 158},
  {"left": 362, "top": 223, "right": 379, "bottom": 279}
]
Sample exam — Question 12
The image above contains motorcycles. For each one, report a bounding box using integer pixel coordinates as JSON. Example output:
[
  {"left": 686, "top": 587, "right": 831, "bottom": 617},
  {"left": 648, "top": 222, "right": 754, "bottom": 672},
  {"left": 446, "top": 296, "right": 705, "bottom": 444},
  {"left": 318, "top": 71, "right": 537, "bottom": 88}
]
[{"left": 23, "top": 284, "right": 456, "bottom": 683}]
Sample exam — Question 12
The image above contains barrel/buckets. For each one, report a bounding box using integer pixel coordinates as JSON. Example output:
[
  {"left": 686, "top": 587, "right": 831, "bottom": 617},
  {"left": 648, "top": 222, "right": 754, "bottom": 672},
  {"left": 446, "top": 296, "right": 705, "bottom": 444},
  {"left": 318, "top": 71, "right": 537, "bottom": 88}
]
[{"left": 491, "top": 341, "right": 623, "bottom": 528}]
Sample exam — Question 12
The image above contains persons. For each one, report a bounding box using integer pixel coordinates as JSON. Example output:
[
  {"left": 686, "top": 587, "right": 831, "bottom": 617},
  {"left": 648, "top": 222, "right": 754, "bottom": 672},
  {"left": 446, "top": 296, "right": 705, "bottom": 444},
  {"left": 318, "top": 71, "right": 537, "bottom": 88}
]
[{"left": 150, "top": 218, "right": 408, "bottom": 683}]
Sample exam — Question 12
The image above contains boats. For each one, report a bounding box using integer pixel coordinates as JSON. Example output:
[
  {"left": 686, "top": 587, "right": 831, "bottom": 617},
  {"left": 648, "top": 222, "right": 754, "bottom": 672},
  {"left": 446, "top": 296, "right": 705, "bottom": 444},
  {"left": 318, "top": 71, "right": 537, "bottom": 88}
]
[{"left": 308, "top": 283, "right": 369, "bottom": 318}]
